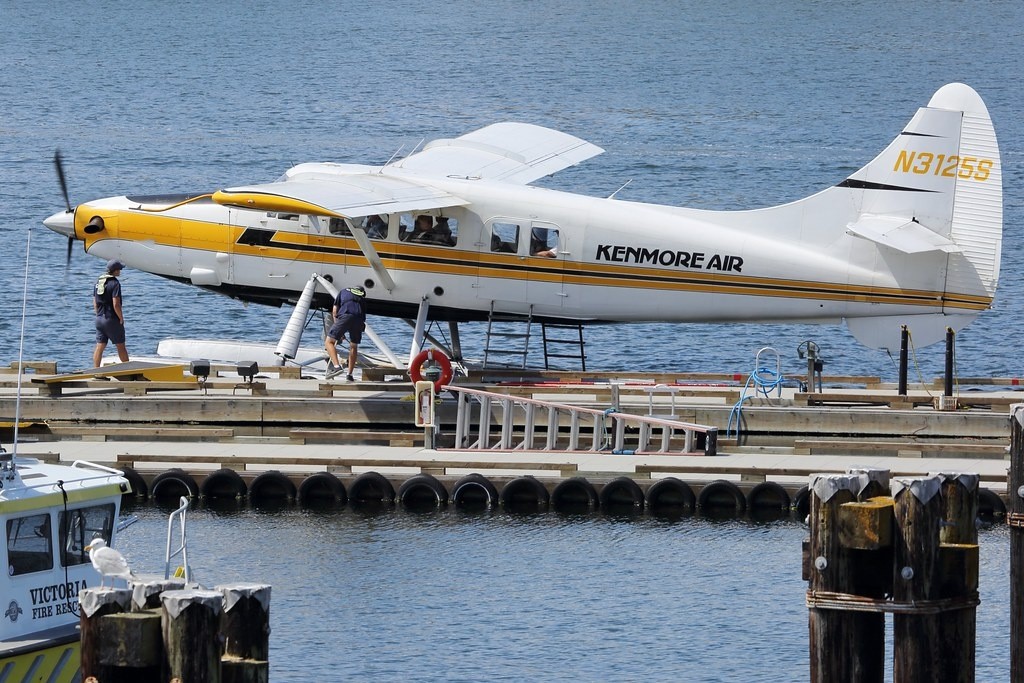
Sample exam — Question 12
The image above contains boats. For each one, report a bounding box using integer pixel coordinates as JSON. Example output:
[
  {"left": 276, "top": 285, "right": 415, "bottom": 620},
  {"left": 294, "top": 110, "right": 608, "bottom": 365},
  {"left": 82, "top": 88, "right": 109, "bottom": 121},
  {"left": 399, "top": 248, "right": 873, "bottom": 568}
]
[{"left": 0, "top": 456, "right": 196, "bottom": 683}]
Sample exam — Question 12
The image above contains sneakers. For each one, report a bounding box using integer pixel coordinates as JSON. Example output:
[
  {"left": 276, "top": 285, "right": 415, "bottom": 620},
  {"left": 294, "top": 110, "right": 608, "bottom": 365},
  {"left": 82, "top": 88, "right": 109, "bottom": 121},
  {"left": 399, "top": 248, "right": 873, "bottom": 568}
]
[
  {"left": 346, "top": 376, "right": 354, "bottom": 382},
  {"left": 326, "top": 367, "right": 344, "bottom": 379}
]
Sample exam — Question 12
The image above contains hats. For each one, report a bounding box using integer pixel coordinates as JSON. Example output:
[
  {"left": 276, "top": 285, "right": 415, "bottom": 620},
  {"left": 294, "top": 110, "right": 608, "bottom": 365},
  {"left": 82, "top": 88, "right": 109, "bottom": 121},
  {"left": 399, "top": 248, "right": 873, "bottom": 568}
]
[
  {"left": 356, "top": 285, "right": 366, "bottom": 297},
  {"left": 106, "top": 259, "right": 125, "bottom": 271}
]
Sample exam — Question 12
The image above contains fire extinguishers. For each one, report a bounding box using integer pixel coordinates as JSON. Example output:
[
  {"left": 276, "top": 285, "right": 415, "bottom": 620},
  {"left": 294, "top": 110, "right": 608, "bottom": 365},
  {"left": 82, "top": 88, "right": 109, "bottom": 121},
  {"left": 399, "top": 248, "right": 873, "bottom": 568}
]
[{"left": 419, "top": 388, "right": 431, "bottom": 424}]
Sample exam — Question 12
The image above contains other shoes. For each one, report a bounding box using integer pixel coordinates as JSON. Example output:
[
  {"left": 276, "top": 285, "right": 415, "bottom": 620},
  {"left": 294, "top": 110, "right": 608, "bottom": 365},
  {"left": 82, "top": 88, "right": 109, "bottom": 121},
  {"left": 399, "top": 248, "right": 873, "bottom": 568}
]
[{"left": 92, "top": 374, "right": 110, "bottom": 381}]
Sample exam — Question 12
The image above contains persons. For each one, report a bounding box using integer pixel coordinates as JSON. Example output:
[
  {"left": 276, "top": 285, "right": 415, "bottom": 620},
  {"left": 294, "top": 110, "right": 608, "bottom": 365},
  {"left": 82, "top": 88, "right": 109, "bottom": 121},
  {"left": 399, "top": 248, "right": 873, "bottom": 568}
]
[
  {"left": 366, "top": 214, "right": 447, "bottom": 246},
  {"left": 531, "top": 230, "right": 559, "bottom": 258},
  {"left": 323, "top": 284, "right": 366, "bottom": 381},
  {"left": 92, "top": 260, "right": 151, "bottom": 382}
]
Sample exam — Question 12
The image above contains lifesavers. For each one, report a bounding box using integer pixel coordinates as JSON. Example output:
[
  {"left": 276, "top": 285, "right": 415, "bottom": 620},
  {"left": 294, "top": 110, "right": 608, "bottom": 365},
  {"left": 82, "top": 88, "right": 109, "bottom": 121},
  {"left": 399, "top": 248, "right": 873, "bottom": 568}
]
[
  {"left": 105, "top": 465, "right": 814, "bottom": 524},
  {"left": 408, "top": 348, "right": 454, "bottom": 394},
  {"left": 979, "top": 489, "right": 1008, "bottom": 529}
]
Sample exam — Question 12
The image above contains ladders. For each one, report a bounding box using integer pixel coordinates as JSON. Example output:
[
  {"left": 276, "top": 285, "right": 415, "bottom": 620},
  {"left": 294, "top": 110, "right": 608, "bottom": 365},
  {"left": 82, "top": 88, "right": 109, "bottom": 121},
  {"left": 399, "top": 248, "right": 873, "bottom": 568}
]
[{"left": 440, "top": 385, "right": 718, "bottom": 456}]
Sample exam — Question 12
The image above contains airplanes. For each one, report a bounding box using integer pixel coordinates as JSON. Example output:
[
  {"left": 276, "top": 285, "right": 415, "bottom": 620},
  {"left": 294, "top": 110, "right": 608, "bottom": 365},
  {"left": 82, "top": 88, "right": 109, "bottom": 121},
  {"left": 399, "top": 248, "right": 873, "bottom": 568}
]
[{"left": 42, "top": 81, "right": 1005, "bottom": 352}]
